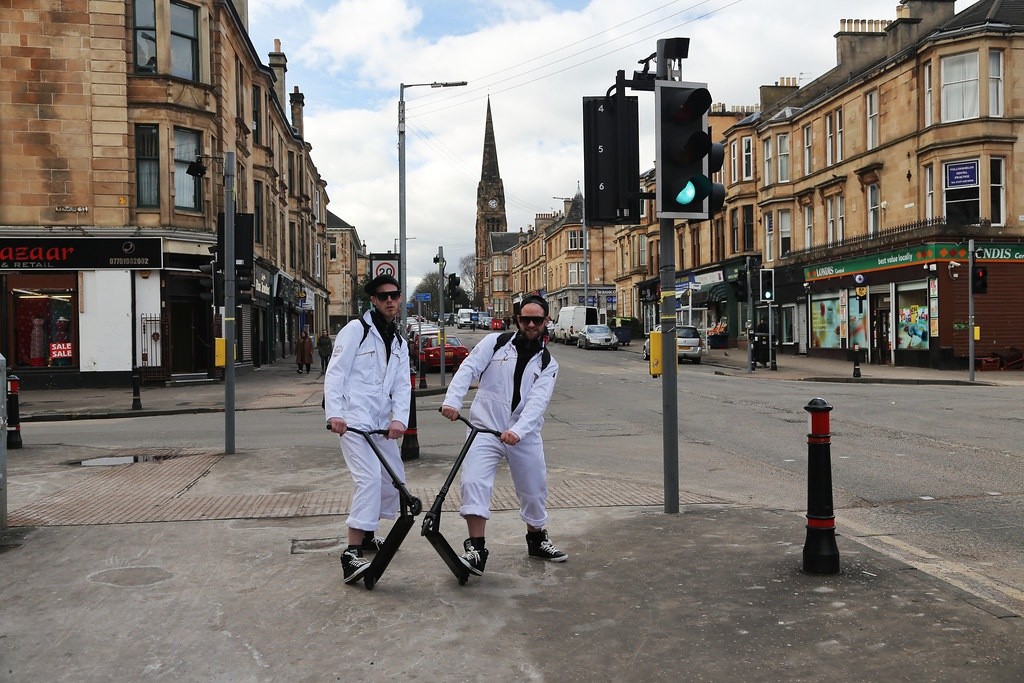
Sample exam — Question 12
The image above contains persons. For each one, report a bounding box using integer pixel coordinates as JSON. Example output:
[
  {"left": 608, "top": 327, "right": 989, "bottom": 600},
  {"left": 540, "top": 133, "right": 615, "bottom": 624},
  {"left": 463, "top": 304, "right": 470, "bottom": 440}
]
[
  {"left": 295, "top": 331, "right": 313, "bottom": 374},
  {"left": 757, "top": 319, "right": 769, "bottom": 333},
  {"left": 441, "top": 296, "right": 568, "bottom": 576},
  {"left": 506, "top": 317, "right": 511, "bottom": 329},
  {"left": 318, "top": 329, "right": 333, "bottom": 373},
  {"left": 324, "top": 274, "right": 412, "bottom": 583}
]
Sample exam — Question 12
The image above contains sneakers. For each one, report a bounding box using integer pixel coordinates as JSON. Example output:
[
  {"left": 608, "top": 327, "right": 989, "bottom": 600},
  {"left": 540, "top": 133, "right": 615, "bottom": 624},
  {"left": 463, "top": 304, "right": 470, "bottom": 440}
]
[
  {"left": 340, "top": 549, "right": 372, "bottom": 584},
  {"left": 526, "top": 529, "right": 568, "bottom": 562},
  {"left": 362, "top": 536, "right": 385, "bottom": 551},
  {"left": 458, "top": 538, "right": 488, "bottom": 575}
]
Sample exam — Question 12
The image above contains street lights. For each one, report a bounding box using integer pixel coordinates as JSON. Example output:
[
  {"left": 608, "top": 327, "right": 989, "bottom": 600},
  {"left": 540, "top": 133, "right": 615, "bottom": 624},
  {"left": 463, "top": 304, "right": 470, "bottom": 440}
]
[
  {"left": 398, "top": 81, "right": 468, "bottom": 345},
  {"left": 656, "top": 37, "right": 692, "bottom": 515},
  {"left": 552, "top": 196, "right": 587, "bottom": 305},
  {"left": 968, "top": 238, "right": 985, "bottom": 382},
  {"left": 394, "top": 236, "right": 417, "bottom": 254},
  {"left": 186, "top": 151, "right": 237, "bottom": 455}
]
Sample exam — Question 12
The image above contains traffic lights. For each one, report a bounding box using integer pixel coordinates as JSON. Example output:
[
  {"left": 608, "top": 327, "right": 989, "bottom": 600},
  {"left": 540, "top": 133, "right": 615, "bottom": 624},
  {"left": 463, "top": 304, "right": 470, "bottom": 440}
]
[
  {"left": 972, "top": 265, "right": 986, "bottom": 294},
  {"left": 759, "top": 269, "right": 774, "bottom": 302},
  {"left": 199, "top": 262, "right": 215, "bottom": 306},
  {"left": 236, "top": 263, "right": 254, "bottom": 306},
  {"left": 654, "top": 78, "right": 713, "bottom": 220},
  {"left": 449, "top": 273, "right": 460, "bottom": 300},
  {"left": 734, "top": 269, "right": 748, "bottom": 302},
  {"left": 708, "top": 125, "right": 726, "bottom": 218}
]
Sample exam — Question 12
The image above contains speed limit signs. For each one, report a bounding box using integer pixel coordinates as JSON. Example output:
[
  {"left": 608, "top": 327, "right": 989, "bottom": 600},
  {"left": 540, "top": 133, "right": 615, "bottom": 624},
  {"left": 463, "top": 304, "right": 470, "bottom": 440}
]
[{"left": 372, "top": 260, "right": 399, "bottom": 282}]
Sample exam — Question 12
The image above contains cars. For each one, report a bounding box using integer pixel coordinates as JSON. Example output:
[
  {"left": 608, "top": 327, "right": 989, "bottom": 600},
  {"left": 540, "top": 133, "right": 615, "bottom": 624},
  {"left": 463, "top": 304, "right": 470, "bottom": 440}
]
[
  {"left": 433, "top": 312, "right": 458, "bottom": 326},
  {"left": 400, "top": 316, "right": 468, "bottom": 374},
  {"left": 477, "top": 311, "right": 507, "bottom": 331},
  {"left": 643, "top": 325, "right": 703, "bottom": 364},
  {"left": 576, "top": 324, "right": 618, "bottom": 351}
]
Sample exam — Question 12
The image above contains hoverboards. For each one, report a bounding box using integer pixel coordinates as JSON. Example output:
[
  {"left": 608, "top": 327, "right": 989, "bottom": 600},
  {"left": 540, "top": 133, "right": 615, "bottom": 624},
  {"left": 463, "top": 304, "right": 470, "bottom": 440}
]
[
  {"left": 421, "top": 406, "right": 505, "bottom": 587},
  {"left": 325, "top": 423, "right": 423, "bottom": 592}
]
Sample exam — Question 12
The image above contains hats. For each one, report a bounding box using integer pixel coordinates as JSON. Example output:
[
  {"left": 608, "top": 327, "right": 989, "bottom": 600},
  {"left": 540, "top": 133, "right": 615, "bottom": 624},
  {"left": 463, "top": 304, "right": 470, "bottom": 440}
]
[
  {"left": 365, "top": 275, "right": 399, "bottom": 294},
  {"left": 520, "top": 296, "right": 549, "bottom": 313}
]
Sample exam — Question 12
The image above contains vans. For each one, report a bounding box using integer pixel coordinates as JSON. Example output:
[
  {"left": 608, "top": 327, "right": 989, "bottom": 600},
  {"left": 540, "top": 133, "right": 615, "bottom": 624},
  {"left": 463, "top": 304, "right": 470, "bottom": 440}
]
[
  {"left": 552, "top": 306, "right": 600, "bottom": 345},
  {"left": 456, "top": 308, "right": 476, "bottom": 329}
]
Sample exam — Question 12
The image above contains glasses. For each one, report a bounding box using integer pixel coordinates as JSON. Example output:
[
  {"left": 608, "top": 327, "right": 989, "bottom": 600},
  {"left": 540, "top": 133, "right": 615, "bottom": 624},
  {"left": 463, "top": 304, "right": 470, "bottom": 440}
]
[
  {"left": 520, "top": 316, "right": 545, "bottom": 326},
  {"left": 373, "top": 291, "right": 401, "bottom": 301}
]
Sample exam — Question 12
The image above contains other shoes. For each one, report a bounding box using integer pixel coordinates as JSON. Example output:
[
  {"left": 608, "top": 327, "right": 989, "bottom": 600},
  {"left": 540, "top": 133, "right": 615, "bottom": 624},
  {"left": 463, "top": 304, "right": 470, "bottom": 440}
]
[{"left": 296, "top": 369, "right": 303, "bottom": 375}]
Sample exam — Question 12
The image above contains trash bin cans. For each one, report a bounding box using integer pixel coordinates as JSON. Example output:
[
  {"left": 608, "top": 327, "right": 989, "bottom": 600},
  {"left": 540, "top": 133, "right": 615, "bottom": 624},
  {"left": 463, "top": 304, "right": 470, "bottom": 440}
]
[
  {"left": 612, "top": 327, "right": 632, "bottom": 345},
  {"left": 749, "top": 333, "right": 776, "bottom": 371}
]
